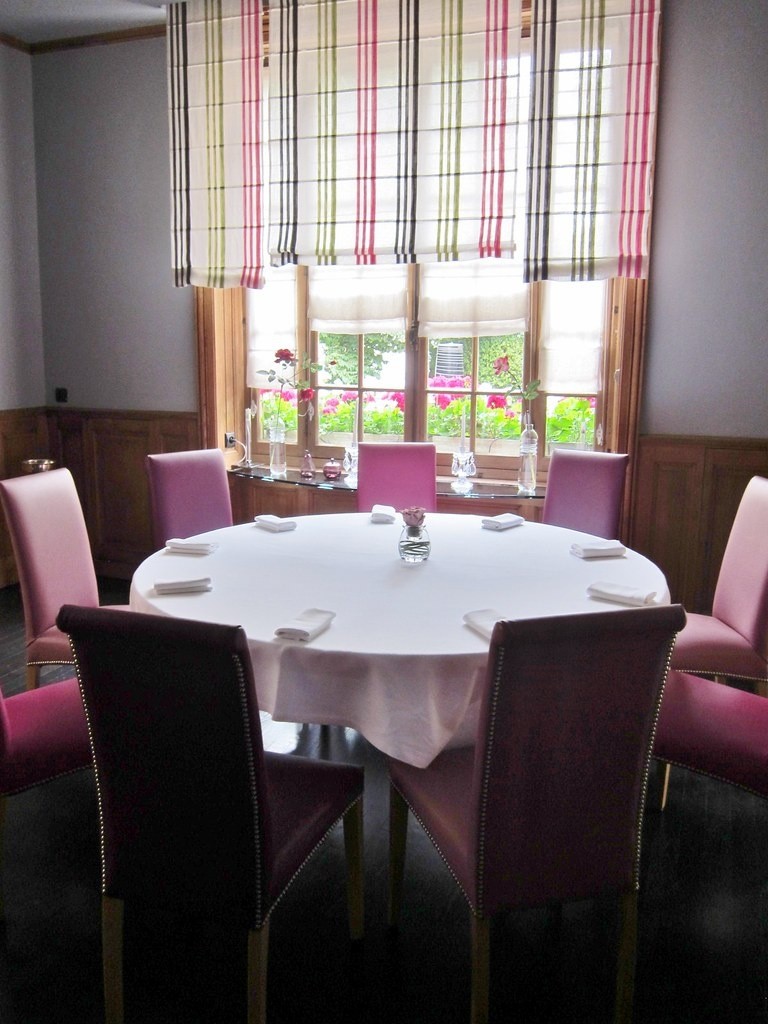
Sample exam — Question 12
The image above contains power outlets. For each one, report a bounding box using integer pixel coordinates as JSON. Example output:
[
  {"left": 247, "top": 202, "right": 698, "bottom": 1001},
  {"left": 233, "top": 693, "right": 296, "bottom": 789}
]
[{"left": 225, "top": 432, "right": 236, "bottom": 448}]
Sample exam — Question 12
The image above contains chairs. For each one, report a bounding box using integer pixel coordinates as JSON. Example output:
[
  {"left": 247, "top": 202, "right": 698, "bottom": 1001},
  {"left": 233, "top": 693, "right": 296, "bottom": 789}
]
[
  {"left": 357, "top": 442, "right": 437, "bottom": 512},
  {"left": 543, "top": 449, "right": 629, "bottom": 541},
  {"left": 0, "top": 466, "right": 100, "bottom": 692},
  {"left": 56, "top": 605, "right": 368, "bottom": 1024},
  {"left": 671, "top": 475, "right": 768, "bottom": 696},
  {"left": 0, "top": 678, "right": 92, "bottom": 820},
  {"left": 145, "top": 447, "right": 233, "bottom": 552},
  {"left": 389, "top": 605, "right": 686, "bottom": 1024}
]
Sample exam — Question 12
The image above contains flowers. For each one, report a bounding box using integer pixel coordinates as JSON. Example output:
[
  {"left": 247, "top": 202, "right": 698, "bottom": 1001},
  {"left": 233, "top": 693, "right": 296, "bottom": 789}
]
[
  {"left": 493, "top": 356, "right": 541, "bottom": 424},
  {"left": 258, "top": 348, "right": 336, "bottom": 417},
  {"left": 398, "top": 506, "right": 425, "bottom": 527}
]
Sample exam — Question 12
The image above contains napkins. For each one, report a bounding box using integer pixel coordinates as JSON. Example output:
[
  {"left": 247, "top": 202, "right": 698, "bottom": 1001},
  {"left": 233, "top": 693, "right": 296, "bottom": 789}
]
[
  {"left": 482, "top": 512, "right": 526, "bottom": 531},
  {"left": 274, "top": 609, "right": 335, "bottom": 641},
  {"left": 369, "top": 505, "right": 399, "bottom": 524},
  {"left": 165, "top": 536, "right": 220, "bottom": 555},
  {"left": 155, "top": 574, "right": 215, "bottom": 594},
  {"left": 584, "top": 582, "right": 659, "bottom": 607},
  {"left": 255, "top": 514, "right": 296, "bottom": 533},
  {"left": 575, "top": 538, "right": 624, "bottom": 558},
  {"left": 460, "top": 610, "right": 514, "bottom": 639}
]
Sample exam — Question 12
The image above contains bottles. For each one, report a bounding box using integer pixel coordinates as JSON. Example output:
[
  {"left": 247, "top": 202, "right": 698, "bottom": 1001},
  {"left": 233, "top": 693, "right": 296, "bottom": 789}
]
[
  {"left": 301, "top": 454, "right": 315, "bottom": 478},
  {"left": 323, "top": 457, "right": 341, "bottom": 478}
]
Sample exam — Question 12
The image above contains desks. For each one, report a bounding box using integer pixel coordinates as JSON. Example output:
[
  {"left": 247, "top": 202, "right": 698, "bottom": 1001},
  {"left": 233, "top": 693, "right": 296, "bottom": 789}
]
[{"left": 130, "top": 512, "right": 671, "bottom": 768}]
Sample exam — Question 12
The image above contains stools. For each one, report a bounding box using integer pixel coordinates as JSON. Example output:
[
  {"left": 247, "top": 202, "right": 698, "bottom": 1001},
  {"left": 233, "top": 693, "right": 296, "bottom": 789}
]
[{"left": 648, "top": 673, "right": 768, "bottom": 814}]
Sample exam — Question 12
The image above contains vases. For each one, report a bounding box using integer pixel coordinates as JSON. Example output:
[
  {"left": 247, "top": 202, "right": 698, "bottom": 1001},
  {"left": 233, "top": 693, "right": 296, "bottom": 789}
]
[
  {"left": 268, "top": 415, "right": 287, "bottom": 475},
  {"left": 398, "top": 525, "right": 431, "bottom": 563},
  {"left": 518, "top": 424, "right": 538, "bottom": 491}
]
